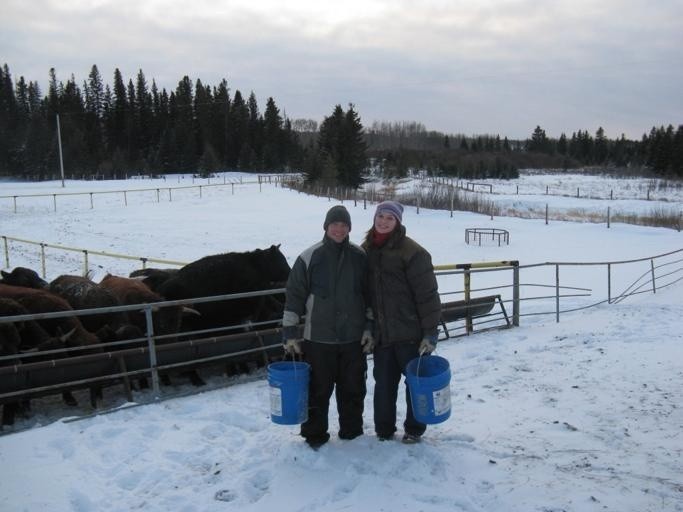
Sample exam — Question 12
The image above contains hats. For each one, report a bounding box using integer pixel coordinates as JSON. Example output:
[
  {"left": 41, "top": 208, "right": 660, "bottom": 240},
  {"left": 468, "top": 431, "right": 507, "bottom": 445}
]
[
  {"left": 324, "top": 205, "right": 351, "bottom": 232},
  {"left": 377, "top": 201, "right": 404, "bottom": 223}
]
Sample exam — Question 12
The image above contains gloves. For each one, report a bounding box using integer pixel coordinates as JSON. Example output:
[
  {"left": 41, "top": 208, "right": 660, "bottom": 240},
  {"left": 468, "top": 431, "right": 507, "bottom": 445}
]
[
  {"left": 361, "top": 330, "right": 374, "bottom": 353},
  {"left": 283, "top": 337, "right": 300, "bottom": 353},
  {"left": 418, "top": 337, "right": 435, "bottom": 356}
]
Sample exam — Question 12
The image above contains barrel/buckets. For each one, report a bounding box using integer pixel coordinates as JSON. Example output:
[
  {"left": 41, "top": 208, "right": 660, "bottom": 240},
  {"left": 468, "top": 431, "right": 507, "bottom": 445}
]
[
  {"left": 266, "top": 349, "right": 311, "bottom": 424},
  {"left": 406, "top": 346, "right": 450, "bottom": 425}
]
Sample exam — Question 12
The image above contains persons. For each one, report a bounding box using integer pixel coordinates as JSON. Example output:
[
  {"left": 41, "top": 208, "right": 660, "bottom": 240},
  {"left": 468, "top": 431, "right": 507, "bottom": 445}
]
[
  {"left": 356, "top": 200, "right": 442, "bottom": 443},
  {"left": 280, "top": 205, "right": 375, "bottom": 448}
]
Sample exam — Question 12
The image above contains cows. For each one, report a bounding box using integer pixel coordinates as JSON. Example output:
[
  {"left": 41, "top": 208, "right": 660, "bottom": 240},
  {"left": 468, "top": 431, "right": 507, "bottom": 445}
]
[
  {"left": 1, "top": 285, "right": 111, "bottom": 409},
  {"left": 0, "top": 296, "right": 81, "bottom": 406},
  {"left": 1, "top": 319, "right": 32, "bottom": 433},
  {"left": 49, "top": 274, "right": 150, "bottom": 395},
  {"left": 156, "top": 245, "right": 291, "bottom": 386},
  {"left": 95, "top": 274, "right": 201, "bottom": 389},
  {"left": 130, "top": 268, "right": 181, "bottom": 292},
  {"left": 0, "top": 267, "right": 50, "bottom": 290}
]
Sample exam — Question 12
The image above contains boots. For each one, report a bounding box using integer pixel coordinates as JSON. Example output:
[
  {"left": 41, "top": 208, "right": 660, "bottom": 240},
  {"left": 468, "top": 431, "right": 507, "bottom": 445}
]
[
  {"left": 338, "top": 427, "right": 363, "bottom": 439},
  {"left": 377, "top": 432, "right": 420, "bottom": 444},
  {"left": 306, "top": 432, "right": 330, "bottom": 443}
]
